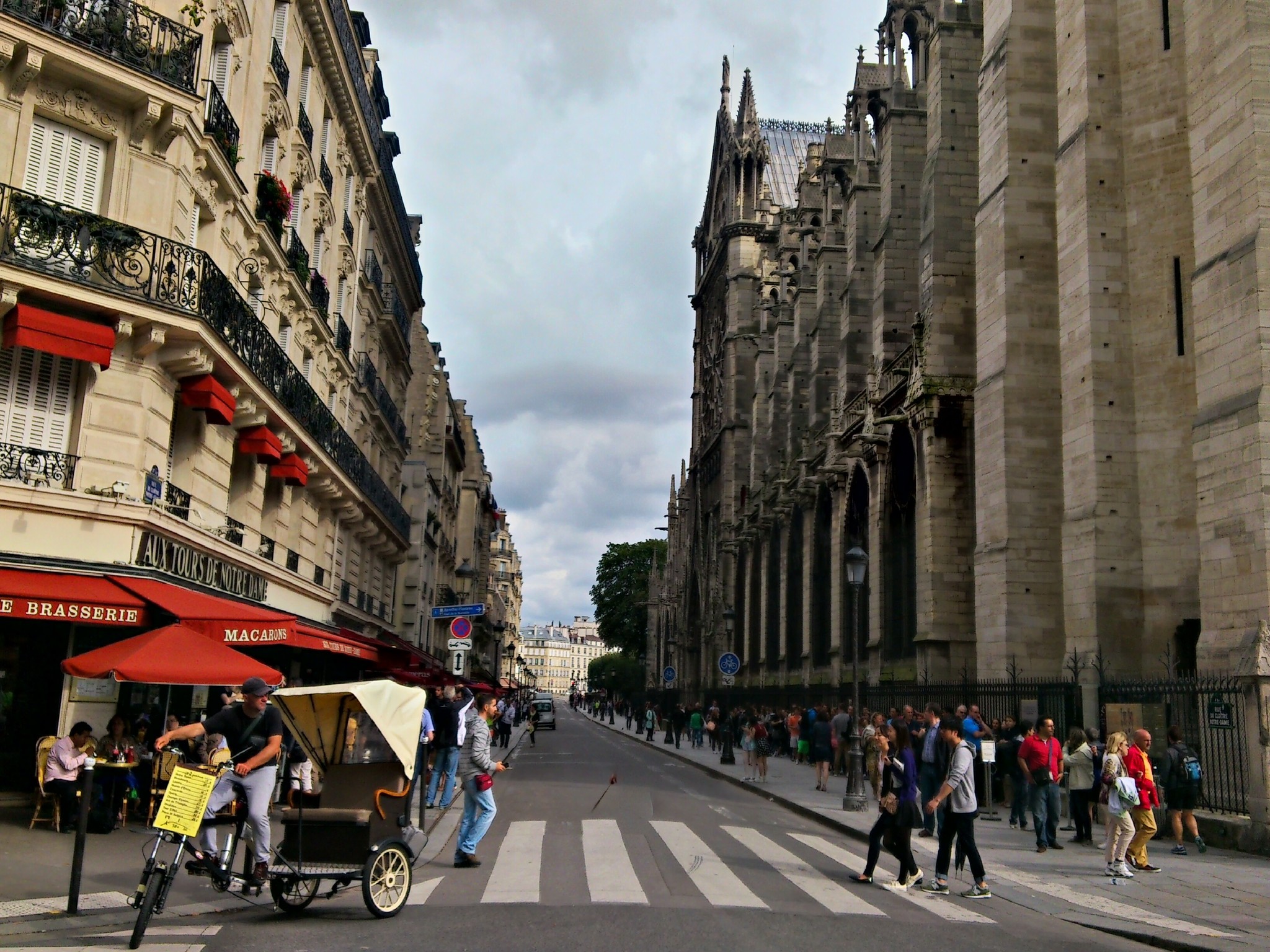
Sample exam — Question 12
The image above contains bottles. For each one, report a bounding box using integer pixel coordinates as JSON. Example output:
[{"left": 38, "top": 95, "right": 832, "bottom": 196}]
[
  {"left": 112, "top": 740, "right": 119, "bottom": 763},
  {"left": 128, "top": 745, "right": 134, "bottom": 763},
  {"left": 123, "top": 740, "right": 130, "bottom": 762}
]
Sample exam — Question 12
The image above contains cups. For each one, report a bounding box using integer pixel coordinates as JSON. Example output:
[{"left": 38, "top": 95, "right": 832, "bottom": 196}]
[{"left": 148, "top": 752, "right": 153, "bottom": 760}]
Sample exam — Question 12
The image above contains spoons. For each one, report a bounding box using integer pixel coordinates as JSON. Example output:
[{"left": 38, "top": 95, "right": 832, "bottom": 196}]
[{"left": 92, "top": 750, "right": 97, "bottom": 756}]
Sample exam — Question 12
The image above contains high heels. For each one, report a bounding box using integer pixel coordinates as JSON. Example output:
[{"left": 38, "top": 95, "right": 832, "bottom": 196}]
[{"left": 815, "top": 783, "right": 827, "bottom": 792}]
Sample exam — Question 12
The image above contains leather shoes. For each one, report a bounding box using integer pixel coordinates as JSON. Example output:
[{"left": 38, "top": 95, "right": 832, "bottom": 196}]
[{"left": 849, "top": 874, "right": 873, "bottom": 883}]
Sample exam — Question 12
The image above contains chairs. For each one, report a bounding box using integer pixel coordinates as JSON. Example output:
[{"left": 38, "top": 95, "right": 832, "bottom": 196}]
[
  {"left": 27, "top": 734, "right": 105, "bottom": 833},
  {"left": 145, "top": 748, "right": 187, "bottom": 830}
]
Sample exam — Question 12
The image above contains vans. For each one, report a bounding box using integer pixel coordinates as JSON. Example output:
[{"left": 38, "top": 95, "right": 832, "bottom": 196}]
[{"left": 529, "top": 692, "right": 556, "bottom": 730}]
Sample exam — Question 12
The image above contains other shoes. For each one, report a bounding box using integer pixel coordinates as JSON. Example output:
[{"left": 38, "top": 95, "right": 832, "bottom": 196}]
[
  {"left": 1037, "top": 845, "right": 1064, "bottom": 853},
  {"left": 530, "top": 742, "right": 535, "bottom": 748},
  {"left": 1067, "top": 836, "right": 1093, "bottom": 847},
  {"left": 739, "top": 775, "right": 768, "bottom": 783},
  {"left": 425, "top": 803, "right": 452, "bottom": 810}
]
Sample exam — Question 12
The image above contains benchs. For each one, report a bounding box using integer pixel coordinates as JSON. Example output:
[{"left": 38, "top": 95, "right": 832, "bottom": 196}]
[{"left": 277, "top": 760, "right": 410, "bottom": 825}]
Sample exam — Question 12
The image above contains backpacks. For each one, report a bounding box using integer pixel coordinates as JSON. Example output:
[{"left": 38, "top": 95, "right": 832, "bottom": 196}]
[{"left": 1170, "top": 744, "right": 1204, "bottom": 785}]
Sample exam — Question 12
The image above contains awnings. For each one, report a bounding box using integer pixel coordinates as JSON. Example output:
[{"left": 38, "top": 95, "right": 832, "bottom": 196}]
[
  {"left": 0, "top": 568, "right": 149, "bottom": 623},
  {"left": 287, "top": 627, "right": 377, "bottom": 662},
  {"left": 340, "top": 627, "right": 410, "bottom": 655},
  {"left": 109, "top": 574, "right": 297, "bottom": 645}
]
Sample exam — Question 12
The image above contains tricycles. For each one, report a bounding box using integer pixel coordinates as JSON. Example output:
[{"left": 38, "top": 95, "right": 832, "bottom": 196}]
[{"left": 130, "top": 677, "right": 427, "bottom": 951}]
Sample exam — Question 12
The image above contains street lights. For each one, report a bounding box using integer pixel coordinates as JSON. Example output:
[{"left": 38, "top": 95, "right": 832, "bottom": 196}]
[
  {"left": 517, "top": 654, "right": 537, "bottom": 688},
  {"left": 609, "top": 670, "right": 616, "bottom": 725},
  {"left": 844, "top": 544, "right": 869, "bottom": 812},
  {"left": 507, "top": 640, "right": 516, "bottom": 688},
  {"left": 570, "top": 672, "right": 606, "bottom": 721}
]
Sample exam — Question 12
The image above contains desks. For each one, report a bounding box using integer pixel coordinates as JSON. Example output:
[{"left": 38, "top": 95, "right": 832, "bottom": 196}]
[{"left": 88, "top": 759, "right": 141, "bottom": 828}]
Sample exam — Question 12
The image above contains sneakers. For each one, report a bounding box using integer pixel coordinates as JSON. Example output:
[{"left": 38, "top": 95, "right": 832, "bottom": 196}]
[
  {"left": 1195, "top": 837, "right": 1207, "bottom": 853},
  {"left": 1171, "top": 845, "right": 1188, "bottom": 856},
  {"left": 1097, "top": 842, "right": 1107, "bottom": 849},
  {"left": 185, "top": 856, "right": 219, "bottom": 872},
  {"left": 960, "top": 883, "right": 992, "bottom": 898},
  {"left": 454, "top": 849, "right": 482, "bottom": 868},
  {"left": 251, "top": 861, "right": 269, "bottom": 886},
  {"left": 920, "top": 879, "right": 950, "bottom": 895},
  {"left": 1124, "top": 854, "right": 1162, "bottom": 873},
  {"left": 1104, "top": 862, "right": 1135, "bottom": 878},
  {"left": 881, "top": 868, "right": 924, "bottom": 892}
]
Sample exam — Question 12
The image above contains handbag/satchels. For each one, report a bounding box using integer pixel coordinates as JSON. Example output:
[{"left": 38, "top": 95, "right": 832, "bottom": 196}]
[
  {"left": 475, "top": 774, "right": 493, "bottom": 791},
  {"left": 1030, "top": 767, "right": 1054, "bottom": 788},
  {"left": 881, "top": 792, "right": 899, "bottom": 815},
  {"left": 1107, "top": 787, "right": 1123, "bottom": 815}
]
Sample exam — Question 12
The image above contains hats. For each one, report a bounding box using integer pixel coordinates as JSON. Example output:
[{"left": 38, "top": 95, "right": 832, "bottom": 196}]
[{"left": 241, "top": 676, "right": 274, "bottom": 697}]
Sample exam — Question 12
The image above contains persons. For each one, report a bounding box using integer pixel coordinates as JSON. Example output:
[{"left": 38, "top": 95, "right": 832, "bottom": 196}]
[
  {"left": 427, "top": 674, "right": 530, "bottom": 748},
  {"left": 43, "top": 721, "right": 95, "bottom": 835},
  {"left": 424, "top": 683, "right": 475, "bottom": 810},
  {"left": 418, "top": 708, "right": 435, "bottom": 745},
  {"left": 156, "top": 676, "right": 282, "bottom": 886},
  {"left": 148, "top": 712, "right": 193, "bottom": 768},
  {"left": 125, "top": 712, "right": 155, "bottom": 813},
  {"left": 95, "top": 714, "right": 142, "bottom": 830},
  {"left": 569, "top": 687, "right": 1202, "bottom": 899},
  {"left": 452, "top": 693, "right": 507, "bottom": 867},
  {"left": 198, "top": 732, "right": 229, "bottom": 764},
  {"left": 282, "top": 735, "right": 313, "bottom": 811},
  {"left": 527, "top": 703, "right": 540, "bottom": 748},
  {"left": 205, "top": 686, "right": 243, "bottom": 721}
]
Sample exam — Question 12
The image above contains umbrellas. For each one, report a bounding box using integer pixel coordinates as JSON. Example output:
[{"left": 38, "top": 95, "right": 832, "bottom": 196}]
[{"left": 59, "top": 624, "right": 285, "bottom": 686}]
